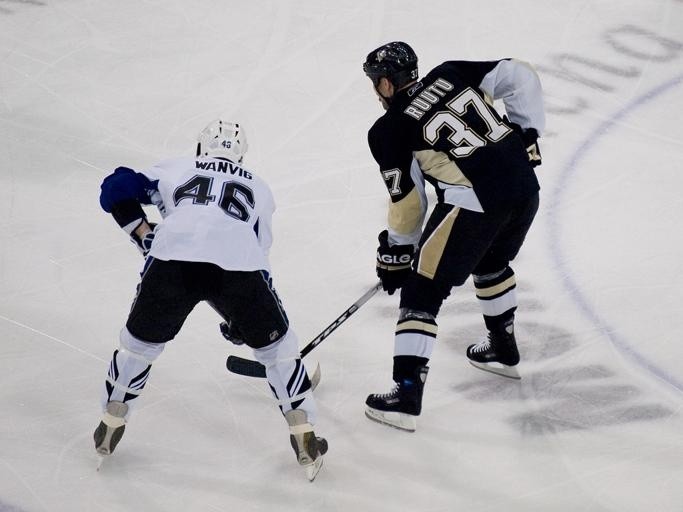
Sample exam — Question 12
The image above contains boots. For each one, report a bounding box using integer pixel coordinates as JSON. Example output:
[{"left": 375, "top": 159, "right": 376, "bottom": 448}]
[
  {"left": 94, "top": 400, "right": 129, "bottom": 457},
  {"left": 286, "top": 409, "right": 328, "bottom": 464},
  {"left": 365, "top": 366, "right": 430, "bottom": 415},
  {"left": 466, "top": 317, "right": 521, "bottom": 366}
]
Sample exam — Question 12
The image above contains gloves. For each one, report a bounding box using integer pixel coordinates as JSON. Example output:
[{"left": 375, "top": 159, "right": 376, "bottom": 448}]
[
  {"left": 218, "top": 321, "right": 243, "bottom": 346},
  {"left": 376, "top": 232, "right": 420, "bottom": 294}
]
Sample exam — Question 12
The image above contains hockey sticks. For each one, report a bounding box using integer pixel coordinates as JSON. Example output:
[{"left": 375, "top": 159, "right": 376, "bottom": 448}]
[
  {"left": 227, "top": 281, "right": 384, "bottom": 377},
  {"left": 205, "top": 300, "right": 321, "bottom": 393}
]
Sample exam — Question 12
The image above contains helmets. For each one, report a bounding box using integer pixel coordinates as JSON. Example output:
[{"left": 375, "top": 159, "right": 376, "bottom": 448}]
[
  {"left": 364, "top": 40, "right": 419, "bottom": 95},
  {"left": 198, "top": 118, "right": 249, "bottom": 166}
]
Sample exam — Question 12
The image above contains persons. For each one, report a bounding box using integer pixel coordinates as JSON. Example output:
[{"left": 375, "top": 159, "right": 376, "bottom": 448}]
[
  {"left": 361, "top": 42, "right": 546, "bottom": 416},
  {"left": 93, "top": 119, "right": 328, "bottom": 466}
]
